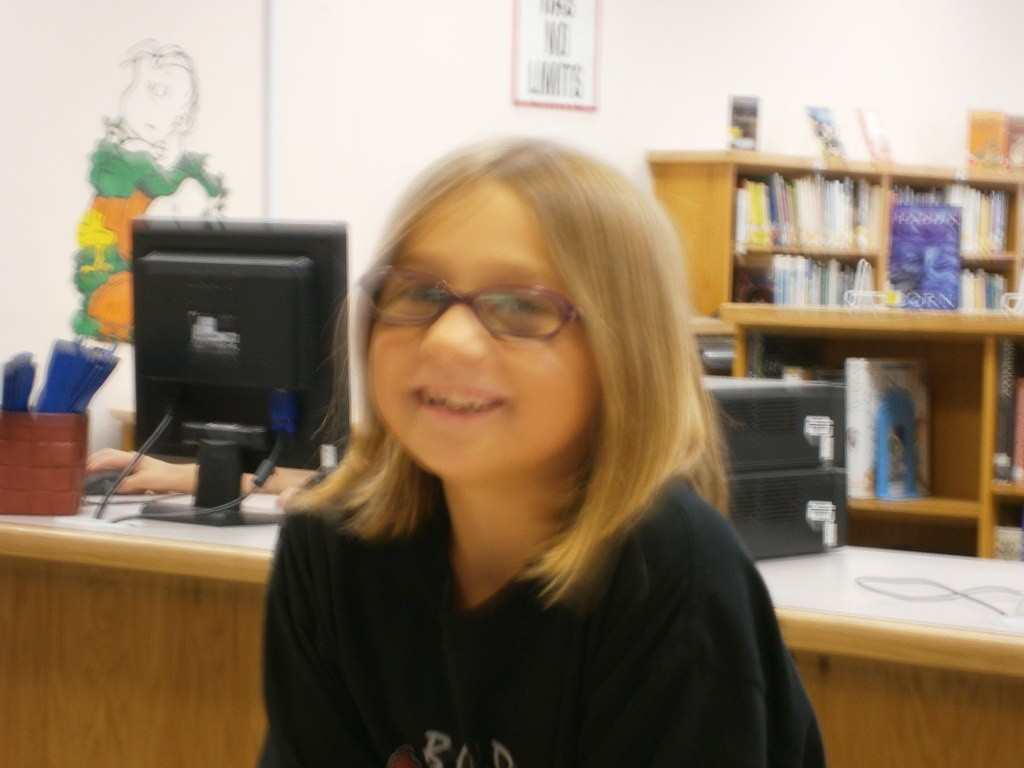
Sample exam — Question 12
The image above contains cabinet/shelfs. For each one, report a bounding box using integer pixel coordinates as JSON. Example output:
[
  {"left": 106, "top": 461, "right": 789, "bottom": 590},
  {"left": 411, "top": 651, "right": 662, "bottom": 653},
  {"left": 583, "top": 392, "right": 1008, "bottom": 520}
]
[
  {"left": 714, "top": 302, "right": 1024, "bottom": 562},
  {"left": 644, "top": 146, "right": 1024, "bottom": 315}
]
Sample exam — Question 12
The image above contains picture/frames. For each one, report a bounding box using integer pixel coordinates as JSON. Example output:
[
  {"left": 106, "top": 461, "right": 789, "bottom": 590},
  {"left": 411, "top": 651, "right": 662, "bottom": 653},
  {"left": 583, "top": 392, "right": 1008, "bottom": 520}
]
[{"left": 509, "top": 0, "right": 603, "bottom": 112}]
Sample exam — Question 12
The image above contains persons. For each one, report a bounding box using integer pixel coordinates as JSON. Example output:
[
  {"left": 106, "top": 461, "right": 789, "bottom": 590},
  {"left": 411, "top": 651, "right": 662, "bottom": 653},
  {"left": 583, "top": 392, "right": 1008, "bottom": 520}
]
[
  {"left": 87, "top": 448, "right": 315, "bottom": 494},
  {"left": 254, "top": 141, "right": 828, "bottom": 767}
]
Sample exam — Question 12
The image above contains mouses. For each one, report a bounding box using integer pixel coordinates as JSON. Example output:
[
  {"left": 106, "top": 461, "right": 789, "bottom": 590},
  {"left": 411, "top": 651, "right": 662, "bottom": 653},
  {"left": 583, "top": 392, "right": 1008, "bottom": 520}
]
[{"left": 83, "top": 470, "right": 129, "bottom": 495}]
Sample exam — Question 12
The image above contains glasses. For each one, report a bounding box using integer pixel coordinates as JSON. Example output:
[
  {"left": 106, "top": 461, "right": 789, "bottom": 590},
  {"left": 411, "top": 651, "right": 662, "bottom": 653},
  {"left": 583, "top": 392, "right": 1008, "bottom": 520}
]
[{"left": 355, "top": 264, "right": 581, "bottom": 339}]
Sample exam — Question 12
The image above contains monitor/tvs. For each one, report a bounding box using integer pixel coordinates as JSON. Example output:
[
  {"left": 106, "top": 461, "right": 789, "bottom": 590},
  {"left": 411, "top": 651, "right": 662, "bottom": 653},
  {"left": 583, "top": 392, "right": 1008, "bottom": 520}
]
[{"left": 130, "top": 223, "right": 355, "bottom": 525}]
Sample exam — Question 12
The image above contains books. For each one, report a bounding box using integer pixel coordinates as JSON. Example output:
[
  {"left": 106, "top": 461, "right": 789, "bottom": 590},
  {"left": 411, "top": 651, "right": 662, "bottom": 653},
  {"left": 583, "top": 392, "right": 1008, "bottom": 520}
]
[
  {"left": 736, "top": 171, "right": 1008, "bottom": 310},
  {"left": 783, "top": 355, "right": 927, "bottom": 500},
  {"left": 998, "top": 336, "right": 1024, "bottom": 486}
]
